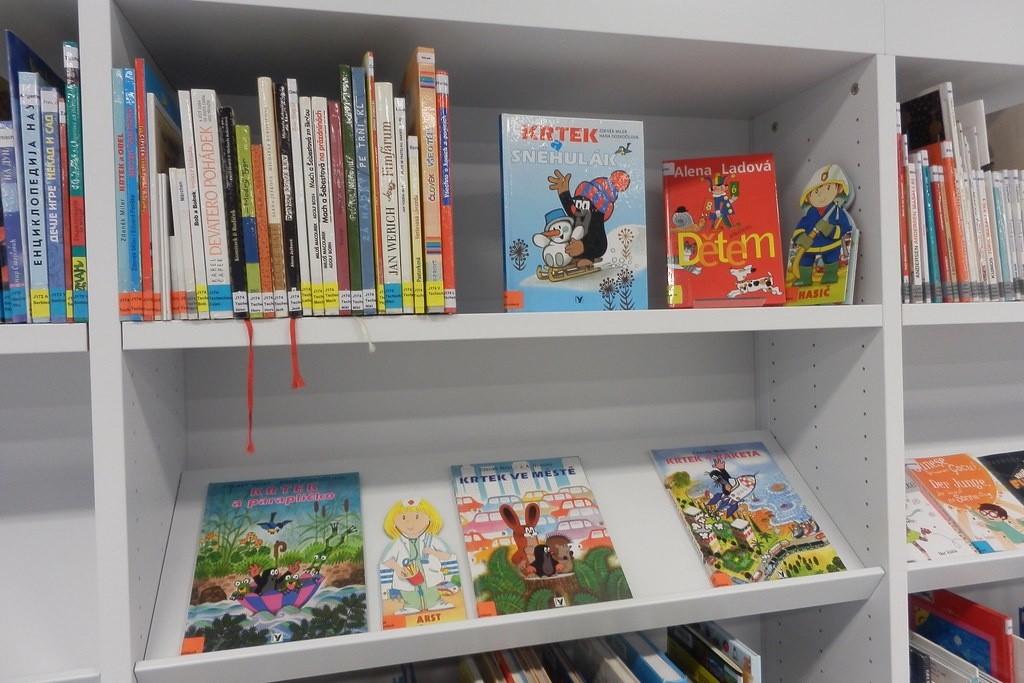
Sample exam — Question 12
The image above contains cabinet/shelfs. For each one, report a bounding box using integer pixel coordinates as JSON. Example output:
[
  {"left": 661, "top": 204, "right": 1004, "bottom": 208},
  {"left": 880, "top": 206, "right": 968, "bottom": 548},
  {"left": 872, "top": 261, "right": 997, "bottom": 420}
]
[{"left": 0, "top": 0, "right": 1024, "bottom": 683}]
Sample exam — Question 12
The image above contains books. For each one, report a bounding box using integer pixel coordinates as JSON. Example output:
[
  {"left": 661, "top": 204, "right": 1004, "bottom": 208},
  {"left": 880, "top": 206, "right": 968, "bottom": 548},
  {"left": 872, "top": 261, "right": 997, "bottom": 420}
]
[
  {"left": 0, "top": 29, "right": 88, "bottom": 325},
  {"left": 112, "top": 46, "right": 457, "bottom": 320},
  {"left": 181, "top": 472, "right": 368, "bottom": 656},
  {"left": 499, "top": 113, "right": 649, "bottom": 313},
  {"left": 661, "top": 153, "right": 786, "bottom": 307},
  {"left": 897, "top": 79, "right": 1023, "bottom": 303},
  {"left": 461, "top": 621, "right": 761, "bottom": 683},
  {"left": 450, "top": 456, "right": 633, "bottom": 618},
  {"left": 905, "top": 451, "right": 1024, "bottom": 683},
  {"left": 650, "top": 442, "right": 848, "bottom": 587}
]
